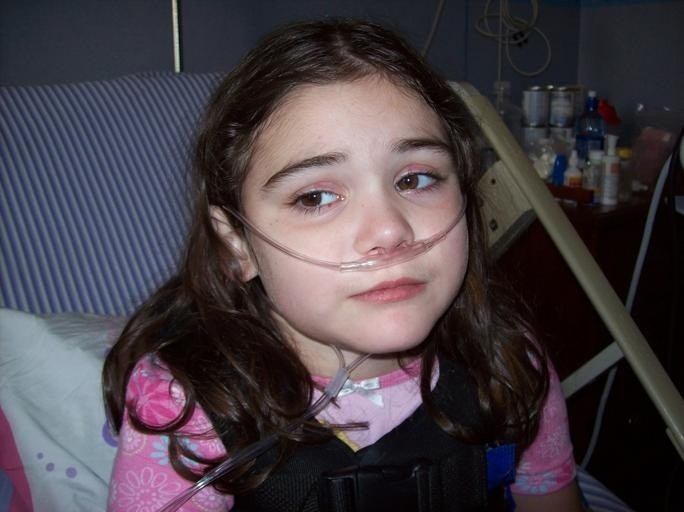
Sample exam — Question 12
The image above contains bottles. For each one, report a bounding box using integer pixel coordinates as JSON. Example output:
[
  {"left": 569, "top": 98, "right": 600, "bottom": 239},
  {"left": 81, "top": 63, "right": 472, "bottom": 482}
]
[
  {"left": 577, "top": 89, "right": 605, "bottom": 160},
  {"left": 551, "top": 151, "right": 604, "bottom": 192},
  {"left": 617, "top": 146, "right": 634, "bottom": 204}
]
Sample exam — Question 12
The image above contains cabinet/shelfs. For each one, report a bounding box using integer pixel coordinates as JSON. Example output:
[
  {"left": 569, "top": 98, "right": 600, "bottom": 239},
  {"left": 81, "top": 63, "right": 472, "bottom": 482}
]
[{"left": 488, "top": 158, "right": 684, "bottom": 512}]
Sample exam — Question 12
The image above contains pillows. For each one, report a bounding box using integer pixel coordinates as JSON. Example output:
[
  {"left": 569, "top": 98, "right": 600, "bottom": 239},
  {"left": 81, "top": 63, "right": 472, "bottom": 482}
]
[{"left": 3, "top": 304, "right": 131, "bottom": 512}]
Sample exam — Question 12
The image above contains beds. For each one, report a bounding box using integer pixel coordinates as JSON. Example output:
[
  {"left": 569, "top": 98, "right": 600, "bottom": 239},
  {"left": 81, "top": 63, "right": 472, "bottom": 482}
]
[{"left": 0, "top": 67, "right": 683, "bottom": 512}]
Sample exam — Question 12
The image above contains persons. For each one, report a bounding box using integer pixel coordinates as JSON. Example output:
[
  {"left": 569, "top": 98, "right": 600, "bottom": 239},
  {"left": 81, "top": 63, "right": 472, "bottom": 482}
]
[{"left": 99, "top": 13, "right": 591, "bottom": 511}]
